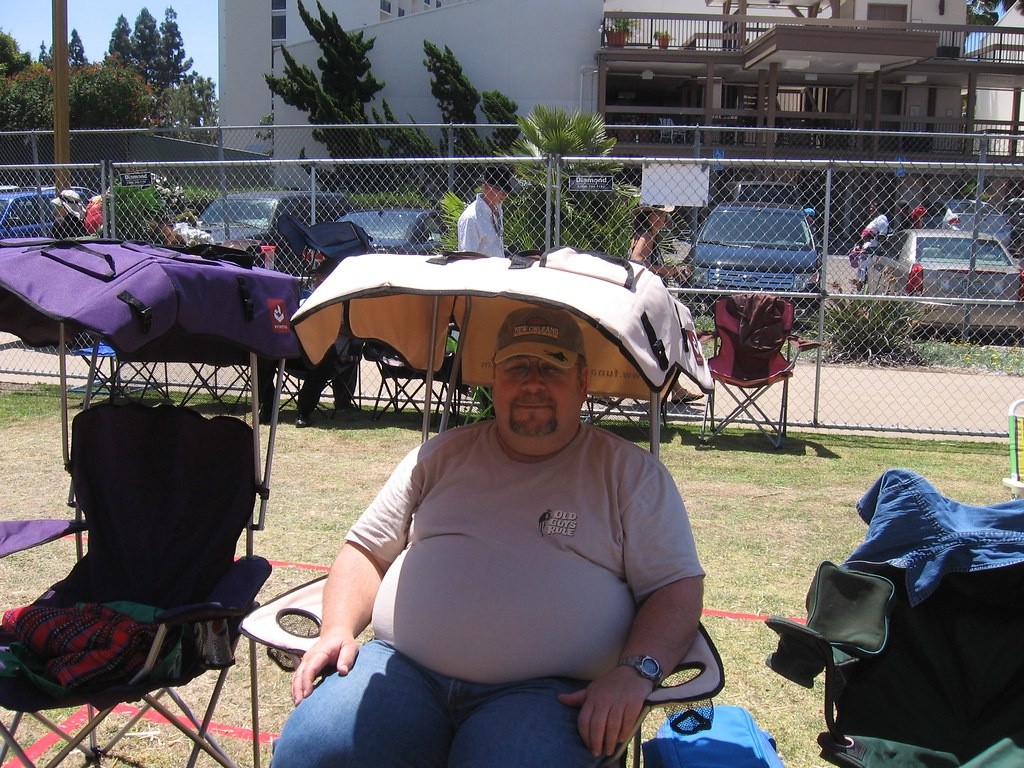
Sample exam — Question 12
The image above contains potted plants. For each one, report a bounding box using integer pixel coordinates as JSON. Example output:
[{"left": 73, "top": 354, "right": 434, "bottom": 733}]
[
  {"left": 599, "top": 15, "right": 641, "bottom": 48},
  {"left": 653, "top": 32, "right": 675, "bottom": 50}
]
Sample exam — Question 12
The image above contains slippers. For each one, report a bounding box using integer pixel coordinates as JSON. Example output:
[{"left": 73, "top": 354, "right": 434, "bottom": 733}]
[{"left": 672, "top": 388, "right": 704, "bottom": 407}]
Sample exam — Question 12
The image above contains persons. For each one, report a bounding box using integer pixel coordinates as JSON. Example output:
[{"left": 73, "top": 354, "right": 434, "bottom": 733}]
[
  {"left": 896, "top": 201, "right": 920, "bottom": 232},
  {"left": 48, "top": 188, "right": 88, "bottom": 240},
  {"left": 458, "top": 166, "right": 523, "bottom": 258},
  {"left": 849, "top": 200, "right": 889, "bottom": 294},
  {"left": 270, "top": 305, "right": 706, "bottom": 768},
  {"left": 630, "top": 199, "right": 692, "bottom": 279}
]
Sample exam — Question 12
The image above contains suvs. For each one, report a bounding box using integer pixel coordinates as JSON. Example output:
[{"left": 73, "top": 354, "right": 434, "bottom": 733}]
[{"left": 0, "top": 186, "right": 99, "bottom": 239}]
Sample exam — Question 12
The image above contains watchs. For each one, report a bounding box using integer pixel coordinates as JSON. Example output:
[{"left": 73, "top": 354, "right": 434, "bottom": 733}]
[{"left": 618, "top": 655, "right": 664, "bottom": 689}]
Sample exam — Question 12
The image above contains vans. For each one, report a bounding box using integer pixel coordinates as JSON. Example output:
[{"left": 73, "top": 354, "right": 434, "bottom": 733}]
[{"left": 198, "top": 190, "right": 348, "bottom": 252}]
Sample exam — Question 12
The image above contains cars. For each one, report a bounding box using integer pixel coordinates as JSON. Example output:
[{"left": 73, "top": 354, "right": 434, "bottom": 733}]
[
  {"left": 915, "top": 198, "right": 1010, "bottom": 246},
  {"left": 1002, "top": 197, "right": 1024, "bottom": 249},
  {"left": 864, "top": 228, "right": 1024, "bottom": 341},
  {"left": 680, "top": 200, "right": 822, "bottom": 313},
  {"left": 697, "top": 179, "right": 800, "bottom": 229},
  {"left": 335, "top": 209, "right": 446, "bottom": 255}
]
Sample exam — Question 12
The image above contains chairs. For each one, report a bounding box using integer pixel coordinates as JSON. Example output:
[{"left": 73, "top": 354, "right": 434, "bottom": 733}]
[
  {"left": 0, "top": 237, "right": 1024, "bottom": 768},
  {"left": 658, "top": 117, "right": 685, "bottom": 144}
]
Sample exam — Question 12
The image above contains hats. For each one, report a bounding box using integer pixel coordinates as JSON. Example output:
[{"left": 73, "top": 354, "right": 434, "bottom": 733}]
[
  {"left": 478, "top": 167, "right": 523, "bottom": 199},
  {"left": 51, "top": 188, "right": 87, "bottom": 219},
  {"left": 495, "top": 306, "right": 586, "bottom": 370},
  {"left": 631, "top": 196, "right": 676, "bottom": 221}
]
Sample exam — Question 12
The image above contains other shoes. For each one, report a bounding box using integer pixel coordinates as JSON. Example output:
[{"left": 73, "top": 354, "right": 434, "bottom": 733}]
[
  {"left": 258, "top": 399, "right": 271, "bottom": 423},
  {"left": 297, "top": 411, "right": 309, "bottom": 428}
]
